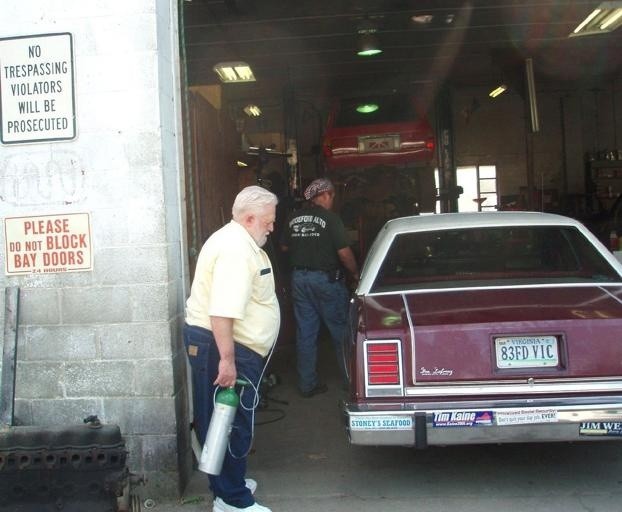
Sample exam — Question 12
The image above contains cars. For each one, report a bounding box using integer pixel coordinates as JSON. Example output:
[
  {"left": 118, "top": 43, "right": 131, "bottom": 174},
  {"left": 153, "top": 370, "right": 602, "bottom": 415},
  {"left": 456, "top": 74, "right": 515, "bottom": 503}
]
[
  {"left": 320, "top": 95, "right": 435, "bottom": 165},
  {"left": 346, "top": 213, "right": 621, "bottom": 447}
]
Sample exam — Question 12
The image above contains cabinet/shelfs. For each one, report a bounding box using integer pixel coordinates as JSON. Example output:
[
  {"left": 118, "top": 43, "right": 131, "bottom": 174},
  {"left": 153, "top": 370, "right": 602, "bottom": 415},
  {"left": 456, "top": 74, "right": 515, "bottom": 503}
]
[{"left": 584, "top": 159, "right": 622, "bottom": 217}]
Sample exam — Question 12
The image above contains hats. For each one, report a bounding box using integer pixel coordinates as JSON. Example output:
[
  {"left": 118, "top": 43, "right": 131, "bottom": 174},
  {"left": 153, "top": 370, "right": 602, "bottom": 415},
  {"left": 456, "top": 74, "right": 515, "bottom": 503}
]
[{"left": 304, "top": 177, "right": 336, "bottom": 200}]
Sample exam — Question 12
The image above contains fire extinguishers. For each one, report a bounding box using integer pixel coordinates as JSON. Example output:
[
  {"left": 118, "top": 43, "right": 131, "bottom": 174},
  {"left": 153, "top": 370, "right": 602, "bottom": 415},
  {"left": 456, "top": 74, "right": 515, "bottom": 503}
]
[{"left": 197, "top": 378, "right": 249, "bottom": 477}]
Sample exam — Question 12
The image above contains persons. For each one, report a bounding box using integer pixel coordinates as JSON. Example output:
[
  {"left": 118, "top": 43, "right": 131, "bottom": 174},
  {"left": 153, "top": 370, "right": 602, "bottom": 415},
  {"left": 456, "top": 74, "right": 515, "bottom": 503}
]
[
  {"left": 280, "top": 178, "right": 359, "bottom": 397},
  {"left": 184, "top": 185, "right": 281, "bottom": 512}
]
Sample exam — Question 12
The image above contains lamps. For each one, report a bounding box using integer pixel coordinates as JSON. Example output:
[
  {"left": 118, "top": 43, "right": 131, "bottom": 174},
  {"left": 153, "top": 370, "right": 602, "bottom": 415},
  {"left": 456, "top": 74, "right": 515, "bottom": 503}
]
[
  {"left": 567, "top": 1, "right": 622, "bottom": 36},
  {"left": 213, "top": 60, "right": 258, "bottom": 84}
]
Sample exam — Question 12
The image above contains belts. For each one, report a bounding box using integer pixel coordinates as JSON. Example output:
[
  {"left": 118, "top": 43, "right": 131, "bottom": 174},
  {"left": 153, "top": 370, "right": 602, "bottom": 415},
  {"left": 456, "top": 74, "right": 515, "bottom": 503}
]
[{"left": 291, "top": 264, "right": 325, "bottom": 271}]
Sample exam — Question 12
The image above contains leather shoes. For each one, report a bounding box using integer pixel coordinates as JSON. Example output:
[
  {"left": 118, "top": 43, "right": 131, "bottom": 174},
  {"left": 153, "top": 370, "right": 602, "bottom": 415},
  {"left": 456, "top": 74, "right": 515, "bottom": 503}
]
[{"left": 301, "top": 383, "right": 329, "bottom": 397}]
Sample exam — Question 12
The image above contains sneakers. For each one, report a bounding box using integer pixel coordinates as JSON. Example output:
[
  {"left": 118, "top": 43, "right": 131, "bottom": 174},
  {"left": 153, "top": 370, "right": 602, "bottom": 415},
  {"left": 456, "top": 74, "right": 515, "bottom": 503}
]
[{"left": 212, "top": 478, "right": 272, "bottom": 512}]
